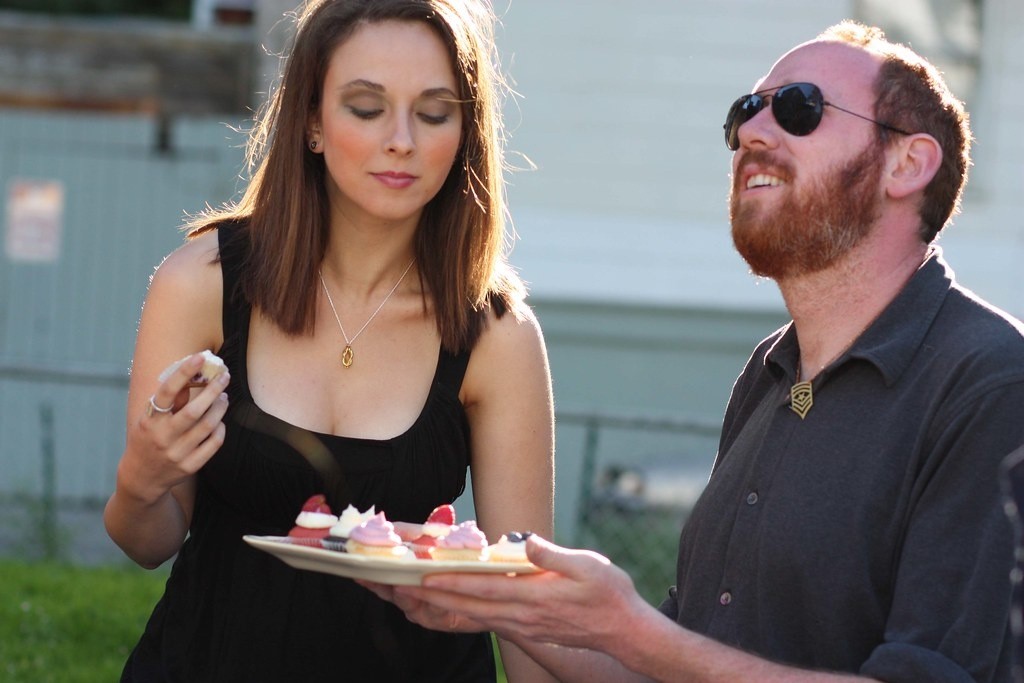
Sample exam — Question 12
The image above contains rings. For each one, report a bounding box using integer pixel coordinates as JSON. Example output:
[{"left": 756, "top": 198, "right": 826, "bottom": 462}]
[{"left": 151, "top": 395, "right": 174, "bottom": 412}]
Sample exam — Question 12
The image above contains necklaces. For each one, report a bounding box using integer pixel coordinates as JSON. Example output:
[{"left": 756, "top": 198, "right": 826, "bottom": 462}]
[
  {"left": 318, "top": 257, "right": 414, "bottom": 367},
  {"left": 797, "top": 322, "right": 867, "bottom": 395}
]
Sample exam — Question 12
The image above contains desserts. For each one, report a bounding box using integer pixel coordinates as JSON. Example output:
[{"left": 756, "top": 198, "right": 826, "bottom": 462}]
[
  {"left": 157, "top": 349, "right": 226, "bottom": 387},
  {"left": 286, "top": 493, "right": 535, "bottom": 564}
]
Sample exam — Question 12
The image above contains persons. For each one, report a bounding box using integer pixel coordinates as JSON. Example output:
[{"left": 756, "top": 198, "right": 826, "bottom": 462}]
[
  {"left": 104, "top": 0, "right": 554, "bottom": 681},
  {"left": 355, "top": 20, "right": 1024, "bottom": 683}
]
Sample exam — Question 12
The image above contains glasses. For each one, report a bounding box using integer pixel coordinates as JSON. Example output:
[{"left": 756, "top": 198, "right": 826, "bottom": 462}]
[{"left": 724, "top": 81, "right": 914, "bottom": 150}]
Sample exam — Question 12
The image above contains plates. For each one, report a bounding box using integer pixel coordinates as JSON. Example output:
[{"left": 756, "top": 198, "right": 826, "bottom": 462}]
[{"left": 242, "top": 534, "right": 545, "bottom": 586}]
